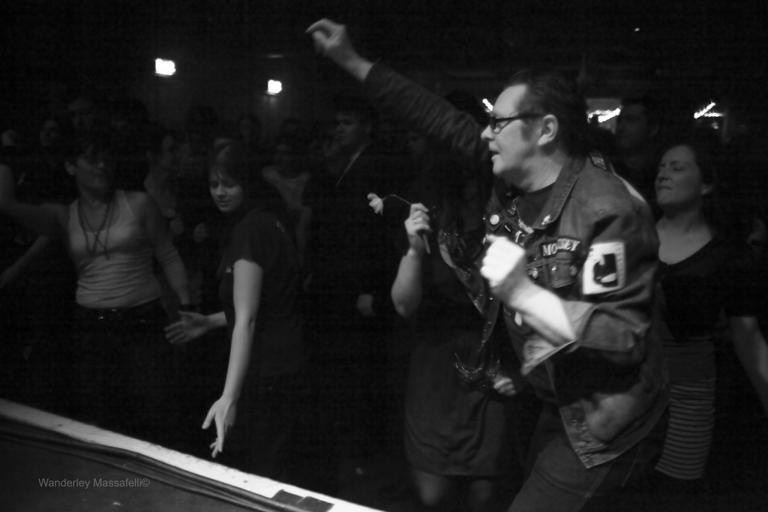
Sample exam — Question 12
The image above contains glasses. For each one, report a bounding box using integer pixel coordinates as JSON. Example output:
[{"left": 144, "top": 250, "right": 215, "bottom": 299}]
[{"left": 489, "top": 113, "right": 546, "bottom": 129}]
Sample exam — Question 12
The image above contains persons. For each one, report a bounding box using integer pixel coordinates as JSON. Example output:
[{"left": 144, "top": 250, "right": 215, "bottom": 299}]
[
  {"left": 608, "top": 95, "right": 767, "bottom": 509},
  {"left": 3, "top": 91, "right": 512, "bottom": 509},
  {"left": 303, "top": 16, "right": 671, "bottom": 511}
]
